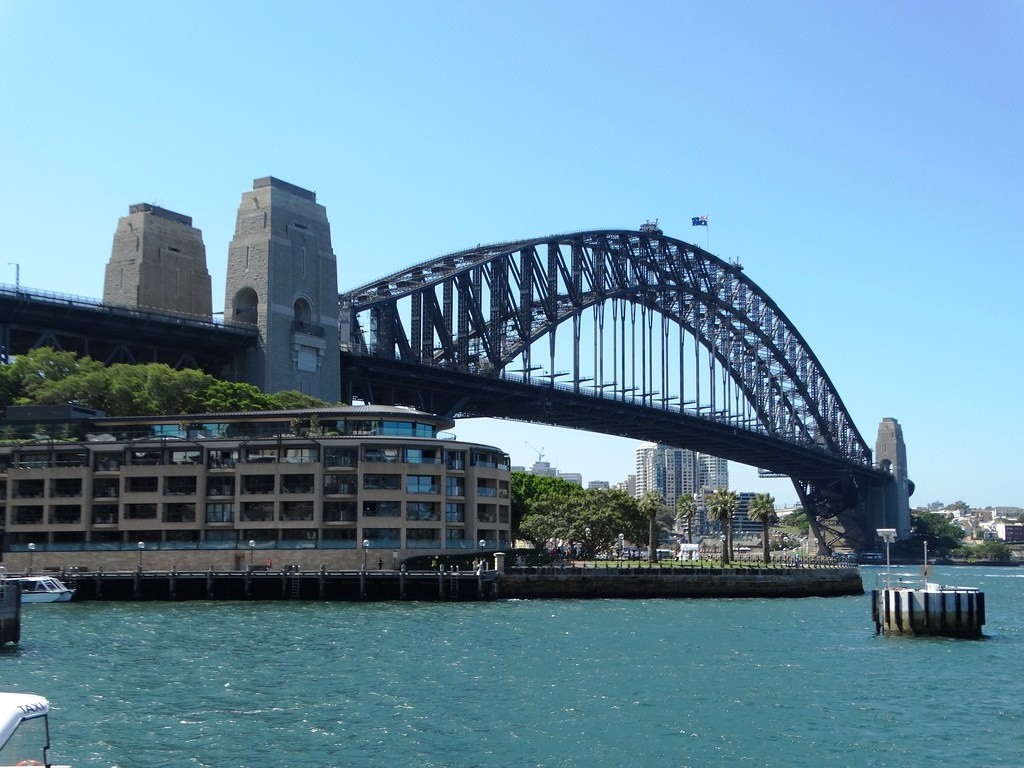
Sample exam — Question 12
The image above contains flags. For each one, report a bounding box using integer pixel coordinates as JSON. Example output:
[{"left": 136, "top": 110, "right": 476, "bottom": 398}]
[{"left": 692, "top": 216, "right": 707, "bottom": 226}]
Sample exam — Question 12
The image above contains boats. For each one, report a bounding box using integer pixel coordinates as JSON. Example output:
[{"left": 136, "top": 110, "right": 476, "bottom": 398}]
[{"left": 0, "top": 565, "right": 77, "bottom": 603}]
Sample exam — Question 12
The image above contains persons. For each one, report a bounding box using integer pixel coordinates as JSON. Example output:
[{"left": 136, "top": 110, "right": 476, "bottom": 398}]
[
  {"left": 267, "top": 559, "right": 272, "bottom": 571},
  {"left": 378, "top": 558, "right": 384, "bottom": 570},
  {"left": 430, "top": 559, "right": 438, "bottom": 570},
  {"left": 473, "top": 557, "right": 487, "bottom": 575},
  {"left": 796, "top": 552, "right": 801, "bottom": 564}
]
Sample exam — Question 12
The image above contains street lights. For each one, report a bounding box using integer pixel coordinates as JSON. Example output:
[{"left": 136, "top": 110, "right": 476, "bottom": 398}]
[
  {"left": 27, "top": 542, "right": 36, "bottom": 575},
  {"left": 478, "top": 539, "right": 486, "bottom": 564},
  {"left": 799, "top": 538, "right": 806, "bottom": 567},
  {"left": 137, "top": 541, "right": 145, "bottom": 571},
  {"left": 249, "top": 539, "right": 256, "bottom": 572},
  {"left": 9, "top": 262, "right": 20, "bottom": 296},
  {"left": 719, "top": 534, "right": 727, "bottom": 567},
  {"left": 813, "top": 538, "right": 819, "bottom": 566},
  {"left": 782, "top": 536, "right": 788, "bottom": 563},
  {"left": 363, "top": 539, "right": 370, "bottom": 570},
  {"left": 618, "top": 533, "right": 624, "bottom": 567}
]
